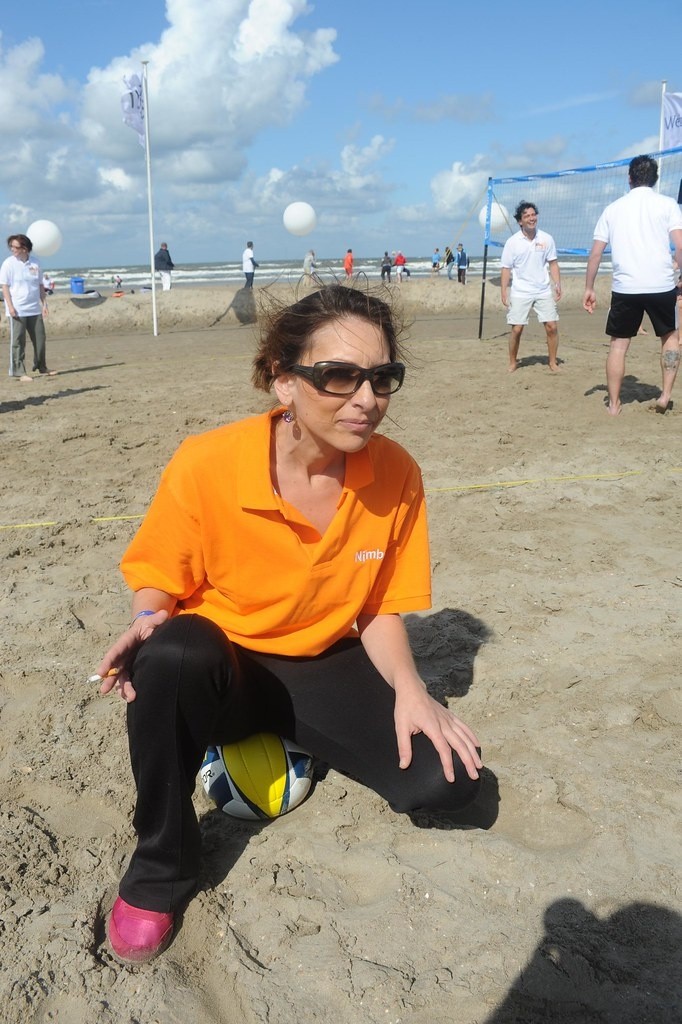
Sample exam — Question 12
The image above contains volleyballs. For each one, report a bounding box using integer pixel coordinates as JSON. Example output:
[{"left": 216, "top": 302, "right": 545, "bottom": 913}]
[
  {"left": 199, "top": 733, "right": 316, "bottom": 820},
  {"left": 392, "top": 251, "right": 396, "bottom": 256}
]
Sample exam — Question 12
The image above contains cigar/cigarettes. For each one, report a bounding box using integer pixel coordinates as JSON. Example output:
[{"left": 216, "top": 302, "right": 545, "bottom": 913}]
[{"left": 86, "top": 668, "right": 118, "bottom": 684}]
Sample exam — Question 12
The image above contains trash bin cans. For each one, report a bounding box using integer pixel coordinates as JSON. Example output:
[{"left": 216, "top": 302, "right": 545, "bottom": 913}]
[{"left": 71, "top": 277, "right": 84, "bottom": 294}]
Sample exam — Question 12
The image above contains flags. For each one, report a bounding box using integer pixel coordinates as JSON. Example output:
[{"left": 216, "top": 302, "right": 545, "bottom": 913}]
[
  {"left": 661, "top": 92, "right": 682, "bottom": 148},
  {"left": 120, "top": 74, "right": 144, "bottom": 145}
]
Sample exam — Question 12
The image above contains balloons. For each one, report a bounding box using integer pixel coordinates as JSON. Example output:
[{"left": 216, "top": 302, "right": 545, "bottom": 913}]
[
  {"left": 477, "top": 202, "right": 509, "bottom": 234},
  {"left": 25, "top": 220, "right": 62, "bottom": 255},
  {"left": 283, "top": 202, "right": 316, "bottom": 238}
]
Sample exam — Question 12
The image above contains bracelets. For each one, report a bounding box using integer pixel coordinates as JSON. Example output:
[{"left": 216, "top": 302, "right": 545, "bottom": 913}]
[
  {"left": 128, "top": 610, "right": 156, "bottom": 631},
  {"left": 555, "top": 283, "right": 560, "bottom": 287}
]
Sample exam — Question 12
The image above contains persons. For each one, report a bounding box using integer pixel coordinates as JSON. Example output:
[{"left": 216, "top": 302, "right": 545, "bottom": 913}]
[
  {"left": 302, "top": 250, "right": 317, "bottom": 287},
  {"left": 97, "top": 268, "right": 484, "bottom": 965},
  {"left": 380, "top": 251, "right": 411, "bottom": 284},
  {"left": 582, "top": 155, "right": 682, "bottom": 416},
  {"left": 499, "top": 199, "right": 563, "bottom": 372},
  {"left": 154, "top": 242, "right": 175, "bottom": 291},
  {"left": 116, "top": 275, "right": 122, "bottom": 289},
  {"left": 0, "top": 235, "right": 57, "bottom": 382},
  {"left": 454, "top": 243, "right": 470, "bottom": 285},
  {"left": 343, "top": 249, "right": 354, "bottom": 281},
  {"left": 431, "top": 248, "right": 441, "bottom": 276},
  {"left": 242, "top": 241, "right": 259, "bottom": 289},
  {"left": 111, "top": 276, "right": 116, "bottom": 288},
  {"left": 444, "top": 247, "right": 455, "bottom": 280}
]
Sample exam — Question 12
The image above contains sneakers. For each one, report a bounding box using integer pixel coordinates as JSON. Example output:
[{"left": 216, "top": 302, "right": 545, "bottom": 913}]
[{"left": 109, "top": 895, "right": 174, "bottom": 966}]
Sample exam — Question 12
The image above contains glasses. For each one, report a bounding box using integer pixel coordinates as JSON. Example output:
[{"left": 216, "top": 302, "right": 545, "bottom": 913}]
[{"left": 289, "top": 361, "right": 405, "bottom": 396}]
